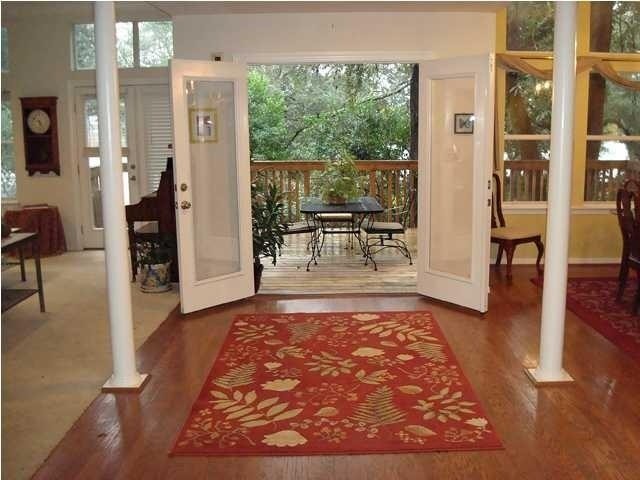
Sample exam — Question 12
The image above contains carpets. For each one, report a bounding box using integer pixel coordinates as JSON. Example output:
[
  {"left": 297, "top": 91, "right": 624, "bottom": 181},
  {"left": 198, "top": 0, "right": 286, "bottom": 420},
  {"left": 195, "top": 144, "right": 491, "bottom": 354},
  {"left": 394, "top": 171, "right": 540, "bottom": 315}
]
[
  {"left": 168, "top": 310, "right": 506, "bottom": 456},
  {"left": 530, "top": 276, "right": 640, "bottom": 368}
]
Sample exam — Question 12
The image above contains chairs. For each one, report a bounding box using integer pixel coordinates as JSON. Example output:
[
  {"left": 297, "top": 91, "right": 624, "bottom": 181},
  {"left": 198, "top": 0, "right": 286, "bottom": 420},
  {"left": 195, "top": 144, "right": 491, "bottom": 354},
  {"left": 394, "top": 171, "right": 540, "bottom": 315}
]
[
  {"left": 315, "top": 213, "right": 355, "bottom": 254},
  {"left": 490, "top": 173, "right": 544, "bottom": 281},
  {"left": 360, "top": 188, "right": 416, "bottom": 266},
  {"left": 615, "top": 179, "right": 640, "bottom": 313}
]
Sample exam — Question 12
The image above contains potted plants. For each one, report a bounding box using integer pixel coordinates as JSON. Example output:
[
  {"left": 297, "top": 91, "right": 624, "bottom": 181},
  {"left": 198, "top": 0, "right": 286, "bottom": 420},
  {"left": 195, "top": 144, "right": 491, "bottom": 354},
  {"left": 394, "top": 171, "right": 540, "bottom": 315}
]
[
  {"left": 127, "top": 236, "right": 173, "bottom": 292},
  {"left": 310, "top": 148, "right": 366, "bottom": 205},
  {"left": 251, "top": 174, "right": 294, "bottom": 293}
]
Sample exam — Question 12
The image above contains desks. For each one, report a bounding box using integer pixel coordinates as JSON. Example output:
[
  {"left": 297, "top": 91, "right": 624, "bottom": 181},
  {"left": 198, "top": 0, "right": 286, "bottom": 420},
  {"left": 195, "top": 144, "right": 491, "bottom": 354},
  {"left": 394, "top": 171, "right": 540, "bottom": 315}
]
[
  {"left": 1, "top": 227, "right": 26, "bottom": 282},
  {"left": 298, "top": 196, "right": 384, "bottom": 272},
  {"left": 5, "top": 205, "right": 68, "bottom": 258},
  {"left": 1, "top": 232, "right": 45, "bottom": 318}
]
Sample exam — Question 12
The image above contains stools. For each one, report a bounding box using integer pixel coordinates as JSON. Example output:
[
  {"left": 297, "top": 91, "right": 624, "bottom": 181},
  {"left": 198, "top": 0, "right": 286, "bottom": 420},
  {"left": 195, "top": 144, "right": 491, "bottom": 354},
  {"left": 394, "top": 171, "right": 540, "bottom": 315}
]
[{"left": 135, "top": 221, "right": 158, "bottom": 270}]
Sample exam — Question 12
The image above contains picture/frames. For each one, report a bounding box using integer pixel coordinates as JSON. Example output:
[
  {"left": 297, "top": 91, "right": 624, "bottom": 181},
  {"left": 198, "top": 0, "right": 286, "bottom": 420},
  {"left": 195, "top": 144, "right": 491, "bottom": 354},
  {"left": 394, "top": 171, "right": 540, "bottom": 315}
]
[
  {"left": 189, "top": 107, "right": 219, "bottom": 143},
  {"left": 453, "top": 113, "right": 473, "bottom": 134}
]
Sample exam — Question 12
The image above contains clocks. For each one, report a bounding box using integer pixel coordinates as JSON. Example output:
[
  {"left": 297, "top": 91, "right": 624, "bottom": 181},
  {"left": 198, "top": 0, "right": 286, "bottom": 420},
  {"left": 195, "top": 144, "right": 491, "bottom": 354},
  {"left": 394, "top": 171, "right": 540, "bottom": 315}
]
[{"left": 18, "top": 96, "right": 60, "bottom": 176}]
